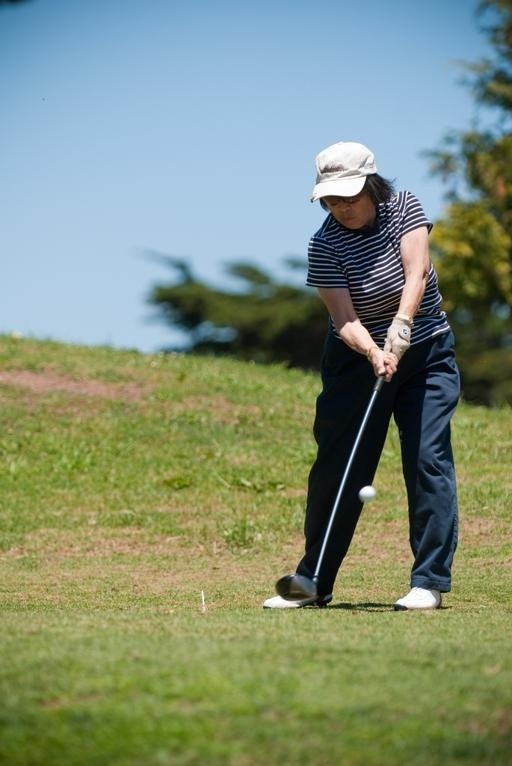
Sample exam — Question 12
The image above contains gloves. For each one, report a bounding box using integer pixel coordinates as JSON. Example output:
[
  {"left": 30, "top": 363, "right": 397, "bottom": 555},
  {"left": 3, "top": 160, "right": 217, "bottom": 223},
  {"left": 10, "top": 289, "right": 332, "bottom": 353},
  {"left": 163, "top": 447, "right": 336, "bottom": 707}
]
[{"left": 384, "top": 314, "right": 414, "bottom": 361}]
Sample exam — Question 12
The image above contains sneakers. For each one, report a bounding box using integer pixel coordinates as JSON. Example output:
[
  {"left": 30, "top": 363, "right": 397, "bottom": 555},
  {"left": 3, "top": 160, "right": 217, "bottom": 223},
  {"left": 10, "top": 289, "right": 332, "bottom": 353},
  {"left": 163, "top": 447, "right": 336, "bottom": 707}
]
[
  {"left": 393, "top": 587, "right": 441, "bottom": 610},
  {"left": 263, "top": 593, "right": 332, "bottom": 608}
]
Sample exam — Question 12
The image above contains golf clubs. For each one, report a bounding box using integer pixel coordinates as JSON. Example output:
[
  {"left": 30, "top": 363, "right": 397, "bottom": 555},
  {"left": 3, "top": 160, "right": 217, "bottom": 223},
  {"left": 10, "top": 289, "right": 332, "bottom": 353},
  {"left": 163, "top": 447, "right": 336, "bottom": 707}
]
[{"left": 277, "top": 374, "right": 388, "bottom": 599}]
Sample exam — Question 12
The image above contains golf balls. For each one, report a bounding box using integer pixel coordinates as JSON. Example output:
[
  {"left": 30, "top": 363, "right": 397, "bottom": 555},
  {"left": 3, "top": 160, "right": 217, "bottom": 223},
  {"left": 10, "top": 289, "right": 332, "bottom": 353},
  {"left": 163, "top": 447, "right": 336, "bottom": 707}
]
[{"left": 358, "top": 485, "right": 378, "bottom": 502}]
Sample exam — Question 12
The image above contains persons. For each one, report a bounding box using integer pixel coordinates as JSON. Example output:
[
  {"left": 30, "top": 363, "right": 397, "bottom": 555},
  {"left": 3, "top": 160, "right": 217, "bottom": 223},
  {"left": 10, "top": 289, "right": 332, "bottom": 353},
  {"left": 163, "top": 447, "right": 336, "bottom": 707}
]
[{"left": 262, "top": 140, "right": 461, "bottom": 610}]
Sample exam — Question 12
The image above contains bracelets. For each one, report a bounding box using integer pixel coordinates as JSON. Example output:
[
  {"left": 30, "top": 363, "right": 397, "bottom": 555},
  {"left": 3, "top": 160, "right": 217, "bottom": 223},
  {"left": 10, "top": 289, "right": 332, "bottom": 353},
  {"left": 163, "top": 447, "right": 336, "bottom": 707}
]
[{"left": 367, "top": 346, "right": 379, "bottom": 360}]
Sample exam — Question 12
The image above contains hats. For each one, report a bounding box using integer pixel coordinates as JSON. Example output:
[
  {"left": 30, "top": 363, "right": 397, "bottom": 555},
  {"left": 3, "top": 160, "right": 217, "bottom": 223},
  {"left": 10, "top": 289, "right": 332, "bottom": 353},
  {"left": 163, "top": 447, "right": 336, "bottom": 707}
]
[{"left": 311, "top": 142, "right": 377, "bottom": 202}]
